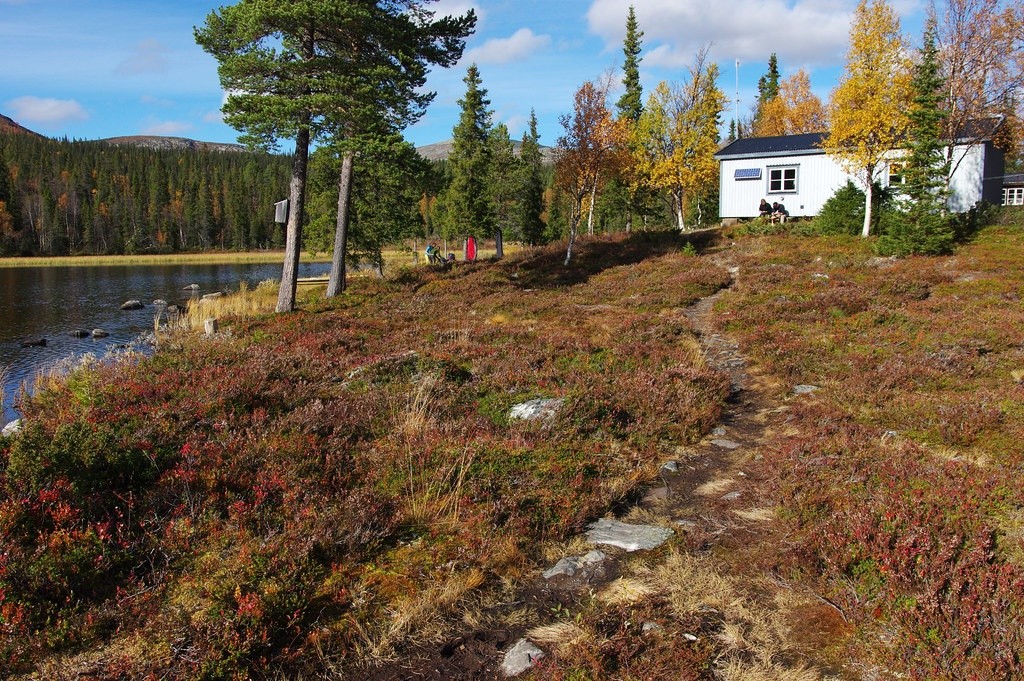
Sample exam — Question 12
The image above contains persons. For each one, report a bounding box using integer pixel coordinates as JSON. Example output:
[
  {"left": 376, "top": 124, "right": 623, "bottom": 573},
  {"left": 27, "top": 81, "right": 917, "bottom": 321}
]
[
  {"left": 756, "top": 199, "right": 773, "bottom": 218},
  {"left": 434, "top": 246, "right": 441, "bottom": 265},
  {"left": 424, "top": 241, "right": 437, "bottom": 267},
  {"left": 770, "top": 202, "right": 790, "bottom": 224}
]
